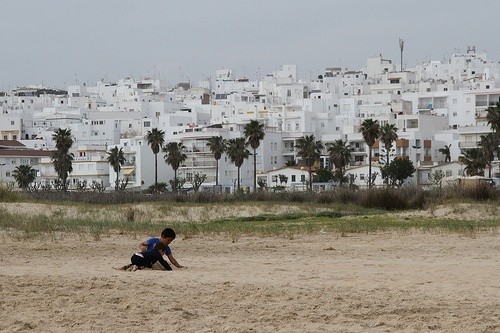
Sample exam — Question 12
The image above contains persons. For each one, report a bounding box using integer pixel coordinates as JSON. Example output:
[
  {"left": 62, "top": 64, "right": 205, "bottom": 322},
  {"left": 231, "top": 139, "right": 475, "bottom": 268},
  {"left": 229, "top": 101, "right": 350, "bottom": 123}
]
[{"left": 127, "top": 228, "right": 188, "bottom": 271}]
